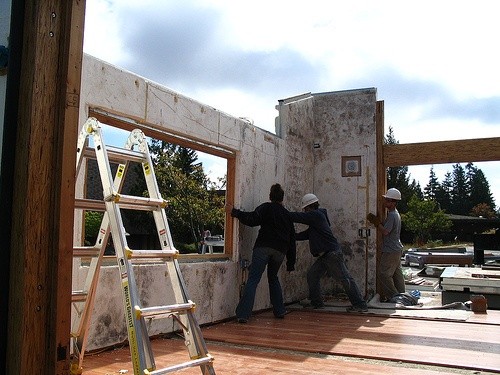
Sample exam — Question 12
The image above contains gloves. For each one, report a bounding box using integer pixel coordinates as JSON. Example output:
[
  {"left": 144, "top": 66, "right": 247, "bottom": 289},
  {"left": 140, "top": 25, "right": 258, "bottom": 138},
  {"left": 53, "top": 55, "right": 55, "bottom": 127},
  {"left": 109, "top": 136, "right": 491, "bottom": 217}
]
[{"left": 231, "top": 208, "right": 240, "bottom": 218}]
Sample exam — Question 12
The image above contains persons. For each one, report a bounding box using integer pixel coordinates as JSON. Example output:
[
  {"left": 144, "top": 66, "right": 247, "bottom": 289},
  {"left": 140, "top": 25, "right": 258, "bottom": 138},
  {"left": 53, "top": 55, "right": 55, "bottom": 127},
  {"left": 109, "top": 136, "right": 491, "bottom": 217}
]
[
  {"left": 288, "top": 193, "right": 366, "bottom": 313},
  {"left": 366, "top": 186, "right": 406, "bottom": 302},
  {"left": 224, "top": 183, "right": 298, "bottom": 322},
  {"left": 202, "top": 230, "right": 211, "bottom": 244}
]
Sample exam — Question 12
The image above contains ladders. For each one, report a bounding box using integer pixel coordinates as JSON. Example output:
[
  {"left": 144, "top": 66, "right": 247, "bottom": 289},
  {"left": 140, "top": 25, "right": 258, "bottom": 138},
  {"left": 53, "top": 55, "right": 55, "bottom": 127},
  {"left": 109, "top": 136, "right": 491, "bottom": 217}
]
[{"left": 70, "top": 117, "right": 216, "bottom": 375}]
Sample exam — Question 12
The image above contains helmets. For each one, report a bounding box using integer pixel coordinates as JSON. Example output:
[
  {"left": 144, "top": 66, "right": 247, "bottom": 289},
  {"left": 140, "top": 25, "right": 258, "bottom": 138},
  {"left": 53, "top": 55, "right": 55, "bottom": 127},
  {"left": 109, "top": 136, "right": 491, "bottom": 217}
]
[
  {"left": 381, "top": 188, "right": 401, "bottom": 200},
  {"left": 301, "top": 194, "right": 319, "bottom": 208}
]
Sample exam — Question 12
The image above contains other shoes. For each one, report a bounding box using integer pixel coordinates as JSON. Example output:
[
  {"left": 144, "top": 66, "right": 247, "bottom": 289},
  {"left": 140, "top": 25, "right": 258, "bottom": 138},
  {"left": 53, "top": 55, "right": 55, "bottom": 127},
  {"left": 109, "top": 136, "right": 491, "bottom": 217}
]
[
  {"left": 236, "top": 316, "right": 248, "bottom": 323},
  {"left": 304, "top": 303, "right": 324, "bottom": 309},
  {"left": 346, "top": 306, "right": 369, "bottom": 313},
  {"left": 276, "top": 306, "right": 287, "bottom": 317}
]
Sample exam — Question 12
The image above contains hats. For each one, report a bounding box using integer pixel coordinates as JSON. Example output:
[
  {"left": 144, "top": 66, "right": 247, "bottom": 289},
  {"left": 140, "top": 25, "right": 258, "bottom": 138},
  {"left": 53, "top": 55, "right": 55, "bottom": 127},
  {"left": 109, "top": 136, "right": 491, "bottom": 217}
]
[{"left": 270, "top": 183, "right": 284, "bottom": 202}]
